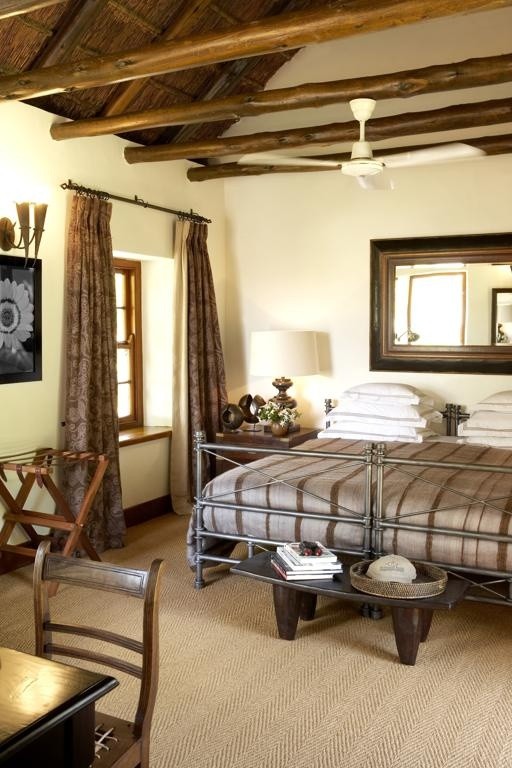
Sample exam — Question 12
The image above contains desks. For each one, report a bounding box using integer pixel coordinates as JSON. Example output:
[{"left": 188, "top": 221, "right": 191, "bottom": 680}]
[{"left": 0, "top": 645, "right": 119, "bottom": 768}]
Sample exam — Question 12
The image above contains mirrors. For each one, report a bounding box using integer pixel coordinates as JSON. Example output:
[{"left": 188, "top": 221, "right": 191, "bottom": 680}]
[{"left": 370, "top": 232, "right": 512, "bottom": 376}]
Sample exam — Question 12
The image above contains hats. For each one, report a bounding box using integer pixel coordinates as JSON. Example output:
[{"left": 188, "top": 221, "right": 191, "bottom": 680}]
[{"left": 365, "top": 553, "right": 417, "bottom": 584}]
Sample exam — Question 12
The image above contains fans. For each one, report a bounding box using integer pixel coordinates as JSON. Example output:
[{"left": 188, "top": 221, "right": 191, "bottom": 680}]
[{"left": 237, "top": 99, "right": 485, "bottom": 189}]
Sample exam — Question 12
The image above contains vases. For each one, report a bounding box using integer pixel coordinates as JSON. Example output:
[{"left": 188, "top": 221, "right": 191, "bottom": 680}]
[{"left": 271, "top": 422, "right": 289, "bottom": 438}]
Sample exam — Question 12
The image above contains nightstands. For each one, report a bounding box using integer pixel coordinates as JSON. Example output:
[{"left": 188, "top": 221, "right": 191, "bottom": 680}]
[{"left": 216, "top": 425, "right": 317, "bottom": 477}]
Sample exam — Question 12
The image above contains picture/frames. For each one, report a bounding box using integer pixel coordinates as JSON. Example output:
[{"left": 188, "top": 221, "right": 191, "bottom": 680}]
[
  {"left": 406, "top": 271, "right": 466, "bottom": 346},
  {"left": 492, "top": 288, "right": 512, "bottom": 346},
  {"left": 0, "top": 255, "right": 43, "bottom": 385}
]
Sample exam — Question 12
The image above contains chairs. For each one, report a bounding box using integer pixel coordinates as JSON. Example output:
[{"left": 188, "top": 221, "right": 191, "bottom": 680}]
[{"left": 32, "top": 541, "right": 166, "bottom": 768}]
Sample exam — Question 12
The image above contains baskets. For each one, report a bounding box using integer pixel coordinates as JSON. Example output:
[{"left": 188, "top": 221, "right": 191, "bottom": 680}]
[{"left": 350, "top": 558, "right": 448, "bottom": 600}]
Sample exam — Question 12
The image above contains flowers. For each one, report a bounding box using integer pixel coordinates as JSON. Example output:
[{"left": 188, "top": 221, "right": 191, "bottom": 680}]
[
  {"left": 258, "top": 401, "right": 303, "bottom": 428},
  {"left": 394, "top": 328, "right": 418, "bottom": 344}
]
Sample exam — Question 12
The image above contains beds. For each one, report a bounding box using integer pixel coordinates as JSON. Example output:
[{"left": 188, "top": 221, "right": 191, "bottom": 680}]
[{"left": 187, "top": 399, "right": 512, "bottom": 607}]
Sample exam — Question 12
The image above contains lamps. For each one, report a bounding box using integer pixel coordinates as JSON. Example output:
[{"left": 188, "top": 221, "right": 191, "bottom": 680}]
[
  {"left": 0, "top": 201, "right": 48, "bottom": 269},
  {"left": 251, "top": 331, "right": 319, "bottom": 432}
]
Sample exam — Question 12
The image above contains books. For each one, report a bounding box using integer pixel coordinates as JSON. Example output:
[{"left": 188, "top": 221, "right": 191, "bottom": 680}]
[{"left": 270, "top": 539, "right": 346, "bottom": 584}]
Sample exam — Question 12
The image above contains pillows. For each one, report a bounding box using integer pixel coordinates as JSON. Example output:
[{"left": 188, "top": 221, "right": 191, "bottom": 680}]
[{"left": 317, "top": 381, "right": 512, "bottom": 448}]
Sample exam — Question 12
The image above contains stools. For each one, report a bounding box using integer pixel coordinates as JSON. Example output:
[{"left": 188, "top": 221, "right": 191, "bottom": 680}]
[{"left": 0, "top": 448, "right": 112, "bottom": 598}]
